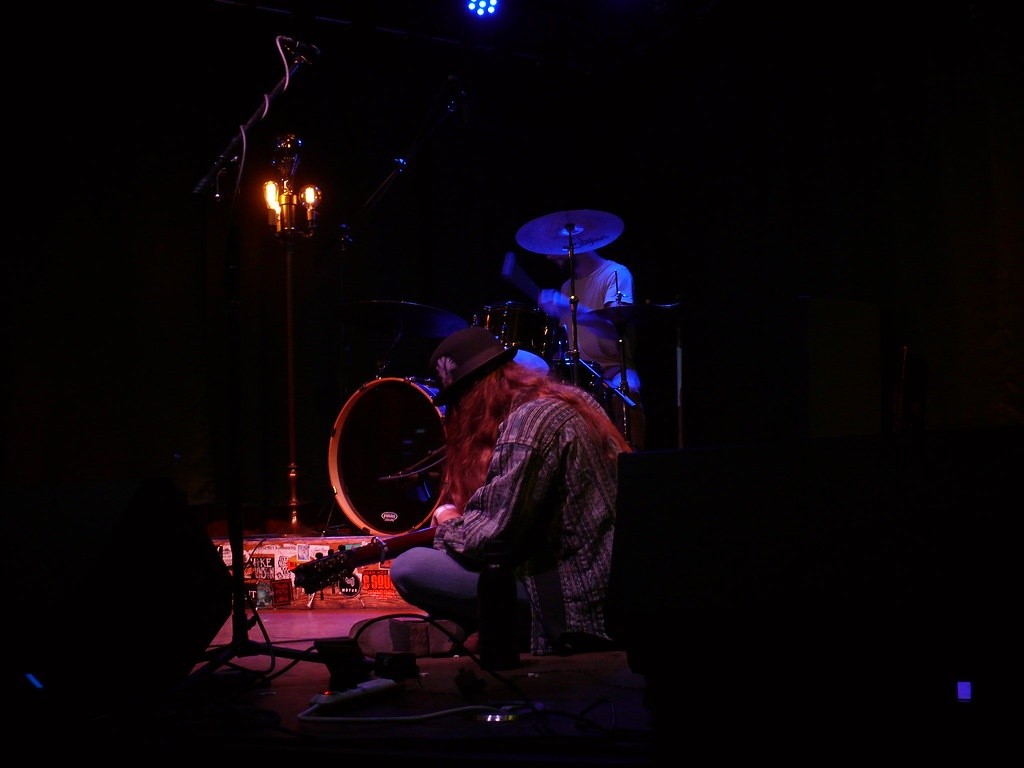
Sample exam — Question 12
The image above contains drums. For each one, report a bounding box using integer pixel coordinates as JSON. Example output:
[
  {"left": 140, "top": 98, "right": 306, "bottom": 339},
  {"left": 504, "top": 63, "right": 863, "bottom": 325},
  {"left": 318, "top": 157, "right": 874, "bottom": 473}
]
[
  {"left": 547, "top": 361, "right": 605, "bottom": 393},
  {"left": 472, "top": 303, "right": 570, "bottom": 361},
  {"left": 327, "top": 377, "right": 451, "bottom": 540}
]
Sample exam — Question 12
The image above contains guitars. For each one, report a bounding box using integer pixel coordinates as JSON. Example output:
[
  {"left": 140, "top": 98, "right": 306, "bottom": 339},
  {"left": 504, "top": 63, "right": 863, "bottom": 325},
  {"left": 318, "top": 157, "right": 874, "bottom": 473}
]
[{"left": 289, "top": 526, "right": 435, "bottom": 609}]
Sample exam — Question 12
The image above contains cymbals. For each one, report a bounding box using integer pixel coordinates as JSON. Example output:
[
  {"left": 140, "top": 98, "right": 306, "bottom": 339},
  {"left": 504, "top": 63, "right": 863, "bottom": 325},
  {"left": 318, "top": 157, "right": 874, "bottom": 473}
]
[
  {"left": 515, "top": 210, "right": 625, "bottom": 256},
  {"left": 349, "top": 300, "right": 467, "bottom": 340},
  {"left": 575, "top": 302, "right": 659, "bottom": 330}
]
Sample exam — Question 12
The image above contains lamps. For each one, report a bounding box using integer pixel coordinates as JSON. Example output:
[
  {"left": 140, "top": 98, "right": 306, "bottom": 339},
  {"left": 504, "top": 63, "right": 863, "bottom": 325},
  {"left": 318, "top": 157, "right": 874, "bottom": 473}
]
[{"left": 257, "top": 133, "right": 324, "bottom": 531}]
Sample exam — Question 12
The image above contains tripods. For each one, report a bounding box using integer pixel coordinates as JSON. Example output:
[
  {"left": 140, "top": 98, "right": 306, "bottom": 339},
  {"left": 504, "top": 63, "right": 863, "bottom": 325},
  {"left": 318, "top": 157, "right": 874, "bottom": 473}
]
[{"left": 190, "top": 65, "right": 375, "bottom": 677}]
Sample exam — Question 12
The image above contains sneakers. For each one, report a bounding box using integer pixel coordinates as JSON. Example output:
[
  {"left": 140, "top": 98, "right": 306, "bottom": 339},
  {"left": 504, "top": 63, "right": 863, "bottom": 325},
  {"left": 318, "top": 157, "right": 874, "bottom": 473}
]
[{"left": 348, "top": 615, "right": 464, "bottom": 659}]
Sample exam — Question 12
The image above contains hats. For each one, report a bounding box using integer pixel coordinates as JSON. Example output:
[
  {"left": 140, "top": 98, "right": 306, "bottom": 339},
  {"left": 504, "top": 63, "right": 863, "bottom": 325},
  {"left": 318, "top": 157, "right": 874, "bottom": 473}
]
[{"left": 430, "top": 326, "right": 519, "bottom": 406}]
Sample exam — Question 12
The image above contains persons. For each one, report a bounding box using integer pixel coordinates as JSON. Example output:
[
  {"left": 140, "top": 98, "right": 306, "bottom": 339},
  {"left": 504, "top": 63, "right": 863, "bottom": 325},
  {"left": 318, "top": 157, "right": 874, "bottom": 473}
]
[
  {"left": 389, "top": 329, "right": 634, "bottom": 657},
  {"left": 537, "top": 249, "right": 646, "bottom": 451}
]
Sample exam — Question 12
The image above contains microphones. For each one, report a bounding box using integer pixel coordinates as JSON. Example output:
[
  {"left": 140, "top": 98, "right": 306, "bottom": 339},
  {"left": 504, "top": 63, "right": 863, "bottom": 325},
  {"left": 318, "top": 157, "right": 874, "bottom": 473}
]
[
  {"left": 447, "top": 75, "right": 466, "bottom": 96},
  {"left": 380, "top": 473, "right": 420, "bottom": 484}
]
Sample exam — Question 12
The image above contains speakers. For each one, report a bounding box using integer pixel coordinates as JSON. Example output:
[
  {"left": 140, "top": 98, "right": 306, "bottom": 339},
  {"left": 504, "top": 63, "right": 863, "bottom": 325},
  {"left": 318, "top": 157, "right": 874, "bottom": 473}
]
[
  {"left": 280, "top": 36, "right": 320, "bottom": 65},
  {"left": 0, "top": 483, "right": 234, "bottom": 730}
]
